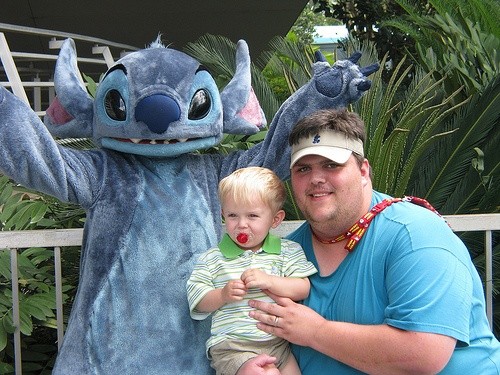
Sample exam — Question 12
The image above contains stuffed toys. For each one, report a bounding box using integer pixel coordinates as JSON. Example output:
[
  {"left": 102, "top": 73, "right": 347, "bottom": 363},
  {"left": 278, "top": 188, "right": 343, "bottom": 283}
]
[{"left": 0, "top": 34, "right": 381, "bottom": 375}]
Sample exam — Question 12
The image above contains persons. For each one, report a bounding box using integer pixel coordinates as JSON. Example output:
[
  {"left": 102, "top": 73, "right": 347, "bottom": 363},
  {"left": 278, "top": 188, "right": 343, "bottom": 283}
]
[
  {"left": 235, "top": 108, "right": 500, "bottom": 375},
  {"left": 186, "top": 166, "right": 318, "bottom": 375}
]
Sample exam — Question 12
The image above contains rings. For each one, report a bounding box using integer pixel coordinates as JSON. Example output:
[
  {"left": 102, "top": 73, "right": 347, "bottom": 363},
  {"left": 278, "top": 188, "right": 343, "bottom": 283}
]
[{"left": 274, "top": 317, "right": 279, "bottom": 328}]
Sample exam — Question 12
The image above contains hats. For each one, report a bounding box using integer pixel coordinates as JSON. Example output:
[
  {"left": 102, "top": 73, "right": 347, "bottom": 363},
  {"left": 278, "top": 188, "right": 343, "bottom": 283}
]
[{"left": 289, "top": 127, "right": 364, "bottom": 170}]
